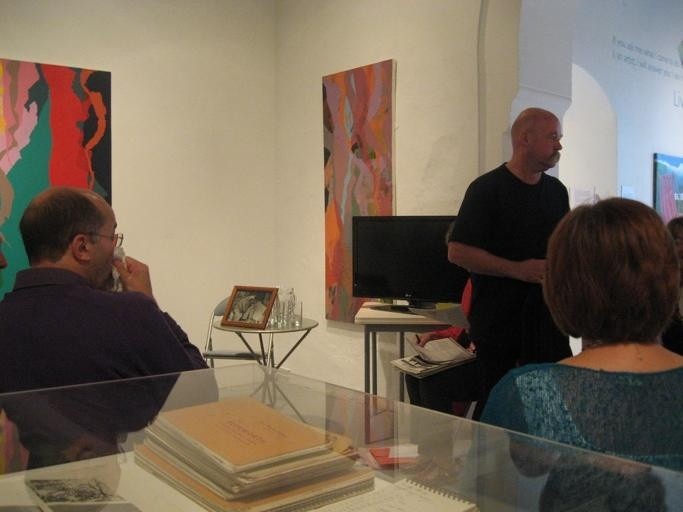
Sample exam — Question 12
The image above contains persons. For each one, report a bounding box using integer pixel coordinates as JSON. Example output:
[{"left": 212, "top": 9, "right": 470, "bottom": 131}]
[
  {"left": 405, "top": 221, "right": 479, "bottom": 489},
  {"left": 448, "top": 107, "right": 573, "bottom": 422},
  {"left": 505, "top": 431, "right": 672, "bottom": 511},
  {"left": 0, "top": 187, "right": 219, "bottom": 391},
  {"left": 0, "top": 234, "right": 8, "bottom": 270},
  {"left": 228, "top": 292, "right": 269, "bottom": 324},
  {"left": 661, "top": 217, "right": 681, "bottom": 355},
  {"left": 478, "top": 196, "right": 681, "bottom": 512},
  {"left": 0, "top": 372, "right": 181, "bottom": 512}
]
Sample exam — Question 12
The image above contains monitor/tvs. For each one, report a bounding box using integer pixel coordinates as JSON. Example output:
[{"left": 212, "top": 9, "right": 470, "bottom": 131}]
[{"left": 352, "top": 215, "right": 469, "bottom": 314}]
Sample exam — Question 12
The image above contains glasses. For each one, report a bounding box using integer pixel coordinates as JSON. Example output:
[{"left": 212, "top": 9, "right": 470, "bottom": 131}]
[{"left": 82, "top": 227, "right": 126, "bottom": 250}]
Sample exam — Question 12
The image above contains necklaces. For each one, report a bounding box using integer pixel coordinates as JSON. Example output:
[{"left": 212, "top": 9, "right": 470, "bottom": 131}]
[{"left": 582, "top": 340, "right": 664, "bottom": 362}]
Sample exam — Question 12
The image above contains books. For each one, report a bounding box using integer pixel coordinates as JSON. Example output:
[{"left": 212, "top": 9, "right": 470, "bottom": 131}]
[
  {"left": 390, "top": 338, "right": 476, "bottom": 380},
  {"left": 135, "top": 397, "right": 479, "bottom": 511},
  {"left": 389, "top": 337, "right": 478, "bottom": 380}
]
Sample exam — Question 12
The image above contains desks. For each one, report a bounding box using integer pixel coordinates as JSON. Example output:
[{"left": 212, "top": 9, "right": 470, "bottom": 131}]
[
  {"left": 1, "top": 361, "right": 683, "bottom": 511},
  {"left": 354, "top": 301, "right": 459, "bottom": 445},
  {"left": 213, "top": 317, "right": 319, "bottom": 424}
]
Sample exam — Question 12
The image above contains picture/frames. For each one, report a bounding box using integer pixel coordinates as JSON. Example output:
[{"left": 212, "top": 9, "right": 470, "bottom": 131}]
[{"left": 219, "top": 284, "right": 279, "bottom": 329}]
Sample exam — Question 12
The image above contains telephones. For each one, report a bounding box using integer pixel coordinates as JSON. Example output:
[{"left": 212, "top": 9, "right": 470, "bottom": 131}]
[{"left": 113, "top": 246, "right": 129, "bottom": 288}]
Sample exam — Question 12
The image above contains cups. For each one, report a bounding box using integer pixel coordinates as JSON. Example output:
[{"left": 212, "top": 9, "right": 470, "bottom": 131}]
[{"left": 267, "top": 288, "right": 304, "bottom": 327}]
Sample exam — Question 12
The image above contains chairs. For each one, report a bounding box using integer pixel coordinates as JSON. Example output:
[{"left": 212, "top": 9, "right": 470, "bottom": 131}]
[{"left": 201, "top": 296, "right": 275, "bottom": 408}]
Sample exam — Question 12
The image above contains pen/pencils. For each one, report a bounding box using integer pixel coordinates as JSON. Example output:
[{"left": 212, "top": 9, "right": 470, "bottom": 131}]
[{"left": 415, "top": 334, "right": 421, "bottom": 343}]
